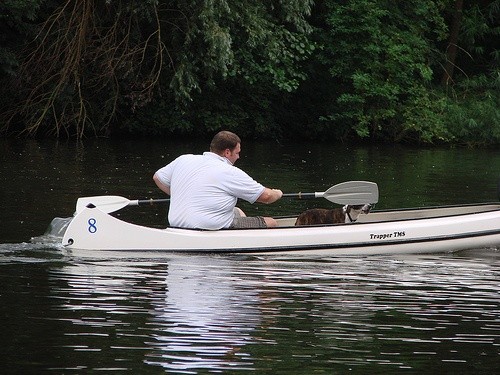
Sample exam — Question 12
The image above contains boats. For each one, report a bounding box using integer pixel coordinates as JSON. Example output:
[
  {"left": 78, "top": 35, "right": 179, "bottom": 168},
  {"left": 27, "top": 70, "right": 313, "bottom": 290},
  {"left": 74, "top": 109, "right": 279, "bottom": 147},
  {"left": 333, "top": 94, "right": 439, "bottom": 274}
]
[{"left": 30, "top": 194, "right": 500, "bottom": 257}]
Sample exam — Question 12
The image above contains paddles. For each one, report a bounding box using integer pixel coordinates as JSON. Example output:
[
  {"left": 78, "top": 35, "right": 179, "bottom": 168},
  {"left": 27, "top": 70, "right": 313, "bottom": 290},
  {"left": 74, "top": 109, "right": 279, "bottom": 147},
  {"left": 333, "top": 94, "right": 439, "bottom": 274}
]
[{"left": 75, "top": 180, "right": 379, "bottom": 214}]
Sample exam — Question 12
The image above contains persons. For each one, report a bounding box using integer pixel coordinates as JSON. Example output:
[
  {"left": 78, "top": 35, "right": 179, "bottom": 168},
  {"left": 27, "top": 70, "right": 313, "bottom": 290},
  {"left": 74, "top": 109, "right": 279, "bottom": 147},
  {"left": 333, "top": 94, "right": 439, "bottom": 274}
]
[{"left": 152, "top": 131, "right": 282, "bottom": 230}]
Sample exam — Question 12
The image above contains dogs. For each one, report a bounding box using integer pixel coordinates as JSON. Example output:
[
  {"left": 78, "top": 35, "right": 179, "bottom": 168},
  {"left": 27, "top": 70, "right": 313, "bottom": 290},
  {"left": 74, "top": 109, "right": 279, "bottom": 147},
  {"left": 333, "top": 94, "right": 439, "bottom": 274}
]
[{"left": 294, "top": 203, "right": 372, "bottom": 227}]
